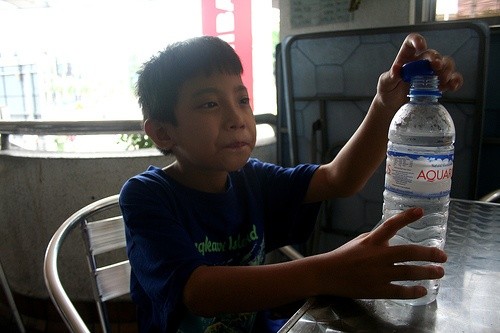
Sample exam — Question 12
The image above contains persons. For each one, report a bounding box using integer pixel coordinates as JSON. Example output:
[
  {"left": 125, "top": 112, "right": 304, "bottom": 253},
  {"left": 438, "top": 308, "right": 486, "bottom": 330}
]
[{"left": 117, "top": 31, "right": 462, "bottom": 333}]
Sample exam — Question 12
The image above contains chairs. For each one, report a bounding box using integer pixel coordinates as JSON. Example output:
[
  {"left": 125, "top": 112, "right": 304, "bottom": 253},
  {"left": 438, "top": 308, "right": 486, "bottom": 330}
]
[{"left": 43, "top": 193, "right": 306, "bottom": 333}]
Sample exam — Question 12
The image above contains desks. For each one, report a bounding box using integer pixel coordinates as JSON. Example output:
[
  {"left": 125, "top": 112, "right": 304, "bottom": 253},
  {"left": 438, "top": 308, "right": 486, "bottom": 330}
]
[{"left": 280, "top": 198, "right": 500, "bottom": 333}]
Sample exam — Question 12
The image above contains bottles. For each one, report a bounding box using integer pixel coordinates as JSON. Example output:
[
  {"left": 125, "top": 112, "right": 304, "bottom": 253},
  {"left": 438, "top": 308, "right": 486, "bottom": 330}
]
[{"left": 380, "top": 58, "right": 456, "bottom": 308}]
